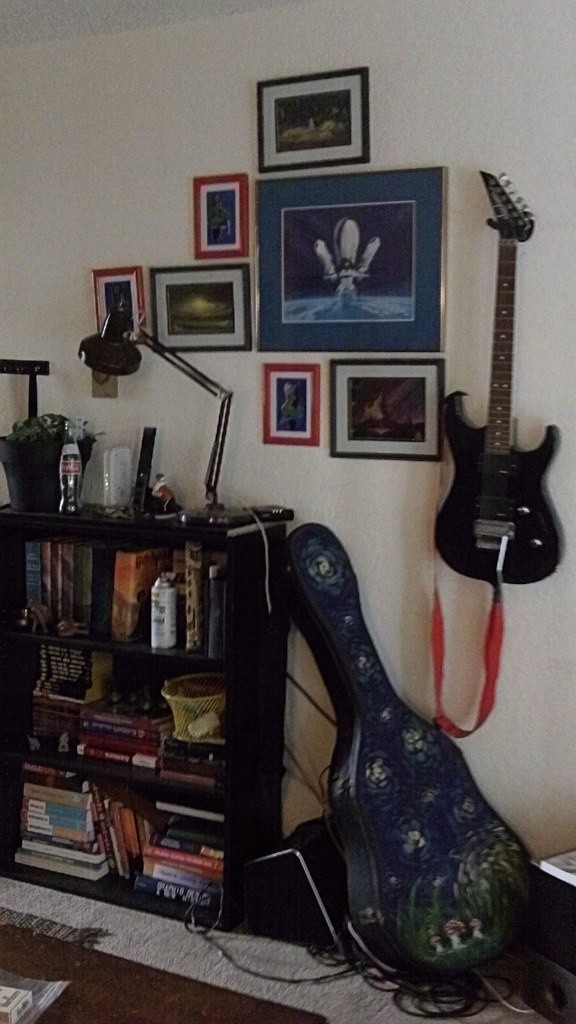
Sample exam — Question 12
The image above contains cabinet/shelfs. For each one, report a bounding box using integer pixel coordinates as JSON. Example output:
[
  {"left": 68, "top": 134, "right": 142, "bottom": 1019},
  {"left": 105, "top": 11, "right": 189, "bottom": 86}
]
[{"left": 1, "top": 505, "right": 291, "bottom": 936}]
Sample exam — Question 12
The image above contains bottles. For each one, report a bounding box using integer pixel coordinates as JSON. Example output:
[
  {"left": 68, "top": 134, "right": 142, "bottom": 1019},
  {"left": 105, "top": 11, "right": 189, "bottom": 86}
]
[
  {"left": 151, "top": 573, "right": 177, "bottom": 650},
  {"left": 59, "top": 420, "right": 83, "bottom": 515}
]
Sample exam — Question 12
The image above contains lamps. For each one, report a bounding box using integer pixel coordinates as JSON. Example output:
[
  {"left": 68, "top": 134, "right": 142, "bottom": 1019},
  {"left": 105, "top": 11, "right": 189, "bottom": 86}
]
[{"left": 78, "top": 302, "right": 260, "bottom": 529}]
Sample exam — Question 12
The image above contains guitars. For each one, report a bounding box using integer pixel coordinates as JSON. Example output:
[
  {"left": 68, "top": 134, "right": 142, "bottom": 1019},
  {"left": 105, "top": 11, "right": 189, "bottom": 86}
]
[{"left": 434, "top": 170, "right": 562, "bottom": 585}]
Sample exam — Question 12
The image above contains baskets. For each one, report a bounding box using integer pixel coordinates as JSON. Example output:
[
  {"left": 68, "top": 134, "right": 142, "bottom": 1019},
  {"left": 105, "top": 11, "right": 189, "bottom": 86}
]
[{"left": 160, "top": 671, "right": 226, "bottom": 743}]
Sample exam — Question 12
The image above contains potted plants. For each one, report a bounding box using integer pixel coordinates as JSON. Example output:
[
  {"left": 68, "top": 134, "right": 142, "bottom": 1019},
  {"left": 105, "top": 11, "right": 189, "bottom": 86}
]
[{"left": 1, "top": 411, "right": 105, "bottom": 516}]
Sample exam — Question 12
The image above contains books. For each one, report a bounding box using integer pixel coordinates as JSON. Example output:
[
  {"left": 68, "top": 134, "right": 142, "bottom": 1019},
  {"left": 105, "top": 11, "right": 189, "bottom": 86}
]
[{"left": 12, "top": 536, "right": 225, "bottom": 915}]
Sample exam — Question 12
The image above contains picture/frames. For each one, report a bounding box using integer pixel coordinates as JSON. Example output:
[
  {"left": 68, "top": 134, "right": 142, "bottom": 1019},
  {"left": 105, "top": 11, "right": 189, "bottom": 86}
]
[
  {"left": 254, "top": 165, "right": 447, "bottom": 354},
  {"left": 149, "top": 262, "right": 254, "bottom": 354},
  {"left": 257, "top": 65, "right": 371, "bottom": 173},
  {"left": 263, "top": 362, "right": 321, "bottom": 447},
  {"left": 330, "top": 357, "right": 444, "bottom": 462},
  {"left": 90, "top": 266, "right": 146, "bottom": 345},
  {"left": 193, "top": 172, "right": 250, "bottom": 261}
]
